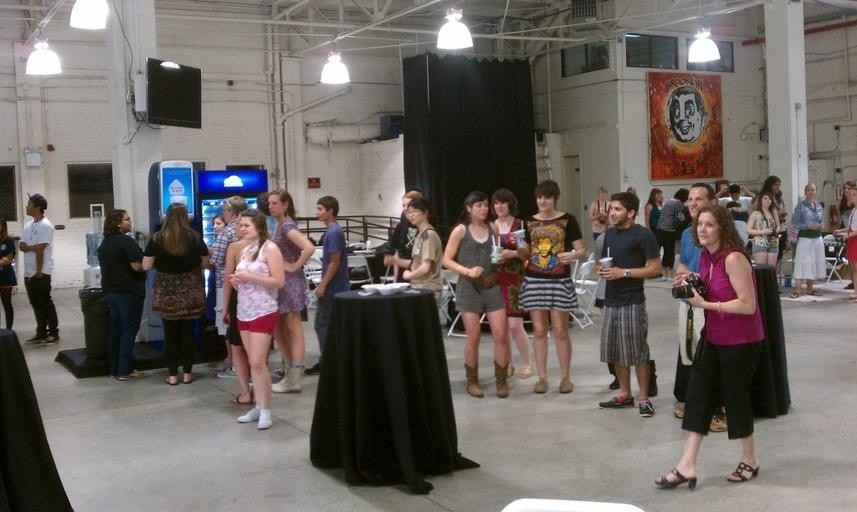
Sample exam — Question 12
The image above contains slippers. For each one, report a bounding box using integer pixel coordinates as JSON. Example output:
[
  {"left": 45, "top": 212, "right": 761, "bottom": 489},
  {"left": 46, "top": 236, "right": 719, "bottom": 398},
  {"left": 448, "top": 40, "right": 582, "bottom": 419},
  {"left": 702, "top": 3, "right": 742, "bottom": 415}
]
[
  {"left": 808, "top": 291, "right": 822, "bottom": 295},
  {"left": 789, "top": 292, "right": 799, "bottom": 298}
]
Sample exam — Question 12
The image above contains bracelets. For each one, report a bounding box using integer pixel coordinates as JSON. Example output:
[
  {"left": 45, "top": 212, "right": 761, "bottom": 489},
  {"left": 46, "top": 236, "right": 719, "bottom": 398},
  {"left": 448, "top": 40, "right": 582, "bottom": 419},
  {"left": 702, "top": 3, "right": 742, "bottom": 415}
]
[
  {"left": 715, "top": 302, "right": 723, "bottom": 311},
  {"left": 465, "top": 269, "right": 470, "bottom": 276}
]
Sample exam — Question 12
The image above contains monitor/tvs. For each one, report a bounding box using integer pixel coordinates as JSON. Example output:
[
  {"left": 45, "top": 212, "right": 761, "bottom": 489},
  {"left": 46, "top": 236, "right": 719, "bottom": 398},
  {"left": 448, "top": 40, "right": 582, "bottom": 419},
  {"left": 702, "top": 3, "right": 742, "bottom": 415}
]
[{"left": 146, "top": 57, "right": 203, "bottom": 130}]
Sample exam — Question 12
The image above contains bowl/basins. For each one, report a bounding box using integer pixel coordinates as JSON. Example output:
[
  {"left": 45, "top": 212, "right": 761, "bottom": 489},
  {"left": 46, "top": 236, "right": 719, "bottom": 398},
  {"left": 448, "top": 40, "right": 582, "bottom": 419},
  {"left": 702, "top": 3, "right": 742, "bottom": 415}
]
[{"left": 361, "top": 282, "right": 410, "bottom": 296}]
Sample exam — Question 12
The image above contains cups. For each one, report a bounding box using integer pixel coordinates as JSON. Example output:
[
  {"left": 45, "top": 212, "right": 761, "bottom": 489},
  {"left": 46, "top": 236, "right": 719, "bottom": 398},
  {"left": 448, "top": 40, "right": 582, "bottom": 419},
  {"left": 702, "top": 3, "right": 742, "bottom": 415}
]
[
  {"left": 514, "top": 229, "right": 527, "bottom": 249},
  {"left": 599, "top": 257, "right": 613, "bottom": 279},
  {"left": 490, "top": 245, "right": 503, "bottom": 264}
]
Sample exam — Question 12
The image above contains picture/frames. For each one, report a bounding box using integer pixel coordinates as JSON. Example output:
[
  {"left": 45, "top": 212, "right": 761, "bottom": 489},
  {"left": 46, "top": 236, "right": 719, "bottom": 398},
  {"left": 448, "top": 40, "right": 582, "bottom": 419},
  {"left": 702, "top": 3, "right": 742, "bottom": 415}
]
[{"left": 646, "top": 71, "right": 724, "bottom": 186}]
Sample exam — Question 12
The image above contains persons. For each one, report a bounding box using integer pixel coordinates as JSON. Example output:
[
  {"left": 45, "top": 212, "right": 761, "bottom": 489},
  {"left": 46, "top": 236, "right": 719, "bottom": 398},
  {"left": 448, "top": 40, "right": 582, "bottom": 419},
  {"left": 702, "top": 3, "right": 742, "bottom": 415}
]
[
  {"left": 383, "top": 197, "right": 443, "bottom": 291},
  {"left": 600, "top": 191, "right": 653, "bottom": 418},
  {"left": 391, "top": 189, "right": 424, "bottom": 284},
  {"left": 591, "top": 233, "right": 658, "bottom": 397},
  {"left": 443, "top": 191, "right": 509, "bottom": 398},
  {"left": 0, "top": 217, "right": 17, "bottom": 330},
  {"left": 510, "top": 181, "right": 585, "bottom": 393},
  {"left": 672, "top": 182, "right": 729, "bottom": 433},
  {"left": 490, "top": 188, "right": 532, "bottom": 380},
  {"left": 219, "top": 189, "right": 350, "bottom": 428},
  {"left": 654, "top": 206, "right": 766, "bottom": 489},
  {"left": 98, "top": 196, "right": 247, "bottom": 385},
  {"left": 19, "top": 191, "right": 61, "bottom": 347},
  {"left": 589, "top": 177, "right": 856, "bottom": 298}
]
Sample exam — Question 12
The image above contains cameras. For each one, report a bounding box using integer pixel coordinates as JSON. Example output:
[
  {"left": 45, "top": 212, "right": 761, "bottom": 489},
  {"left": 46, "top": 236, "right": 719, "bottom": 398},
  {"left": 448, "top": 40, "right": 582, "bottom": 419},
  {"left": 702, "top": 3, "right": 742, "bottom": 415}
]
[{"left": 672, "top": 272, "right": 705, "bottom": 300}]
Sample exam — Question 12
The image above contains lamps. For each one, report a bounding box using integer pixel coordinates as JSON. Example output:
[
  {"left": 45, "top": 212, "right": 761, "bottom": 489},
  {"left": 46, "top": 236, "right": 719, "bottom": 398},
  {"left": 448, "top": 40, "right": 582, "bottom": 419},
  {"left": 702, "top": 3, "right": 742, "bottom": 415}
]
[
  {"left": 320, "top": 37, "right": 351, "bottom": 85},
  {"left": 67, "top": 1, "right": 109, "bottom": 30},
  {"left": 25, "top": 26, "right": 62, "bottom": 76},
  {"left": 436, "top": 0, "right": 474, "bottom": 50},
  {"left": 688, "top": 0, "right": 721, "bottom": 63}
]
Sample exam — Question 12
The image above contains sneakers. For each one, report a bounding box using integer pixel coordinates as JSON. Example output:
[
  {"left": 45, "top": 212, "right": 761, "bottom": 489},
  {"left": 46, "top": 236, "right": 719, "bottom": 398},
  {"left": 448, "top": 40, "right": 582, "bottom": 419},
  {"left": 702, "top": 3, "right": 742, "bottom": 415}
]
[
  {"left": 41, "top": 334, "right": 60, "bottom": 345},
  {"left": 108, "top": 358, "right": 320, "bottom": 430},
  {"left": 26, "top": 336, "right": 42, "bottom": 345},
  {"left": 464, "top": 361, "right": 727, "bottom": 433}
]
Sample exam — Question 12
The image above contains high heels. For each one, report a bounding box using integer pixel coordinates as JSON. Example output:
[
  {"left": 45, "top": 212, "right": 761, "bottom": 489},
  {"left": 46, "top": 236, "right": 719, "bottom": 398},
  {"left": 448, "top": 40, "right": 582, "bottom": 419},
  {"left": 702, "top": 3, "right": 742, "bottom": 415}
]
[
  {"left": 727, "top": 463, "right": 760, "bottom": 482},
  {"left": 654, "top": 468, "right": 697, "bottom": 489}
]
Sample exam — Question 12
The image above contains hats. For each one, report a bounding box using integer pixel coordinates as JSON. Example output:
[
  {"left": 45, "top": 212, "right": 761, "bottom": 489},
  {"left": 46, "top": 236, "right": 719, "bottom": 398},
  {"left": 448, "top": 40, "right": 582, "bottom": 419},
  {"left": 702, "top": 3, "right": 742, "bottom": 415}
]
[{"left": 27, "top": 191, "right": 47, "bottom": 212}]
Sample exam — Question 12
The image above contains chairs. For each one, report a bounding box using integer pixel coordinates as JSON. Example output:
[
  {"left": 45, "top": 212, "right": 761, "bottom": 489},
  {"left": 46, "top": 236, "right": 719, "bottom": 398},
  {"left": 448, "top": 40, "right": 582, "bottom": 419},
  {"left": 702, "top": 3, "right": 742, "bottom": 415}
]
[
  {"left": 304, "top": 244, "right": 394, "bottom": 308},
  {"left": 823, "top": 239, "right": 846, "bottom": 284},
  {"left": 442, "top": 250, "right": 597, "bottom": 338}
]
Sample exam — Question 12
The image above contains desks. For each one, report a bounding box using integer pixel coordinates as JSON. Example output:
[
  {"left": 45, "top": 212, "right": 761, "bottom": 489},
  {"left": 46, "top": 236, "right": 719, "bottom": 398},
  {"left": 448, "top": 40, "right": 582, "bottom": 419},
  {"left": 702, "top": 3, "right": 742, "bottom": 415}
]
[{"left": 310, "top": 288, "right": 478, "bottom": 496}]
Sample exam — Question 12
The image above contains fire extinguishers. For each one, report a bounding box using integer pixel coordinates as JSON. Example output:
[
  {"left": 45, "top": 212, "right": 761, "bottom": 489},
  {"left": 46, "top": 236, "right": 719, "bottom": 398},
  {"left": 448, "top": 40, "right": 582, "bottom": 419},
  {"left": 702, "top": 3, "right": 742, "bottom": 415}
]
[{"left": 830, "top": 205, "right": 837, "bottom": 226}]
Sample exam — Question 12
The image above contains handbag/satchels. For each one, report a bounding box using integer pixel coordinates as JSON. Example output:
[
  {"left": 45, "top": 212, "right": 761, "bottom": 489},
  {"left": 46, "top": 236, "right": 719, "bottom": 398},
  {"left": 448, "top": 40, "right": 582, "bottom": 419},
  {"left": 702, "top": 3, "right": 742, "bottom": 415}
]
[{"left": 787, "top": 224, "right": 800, "bottom": 241}]
